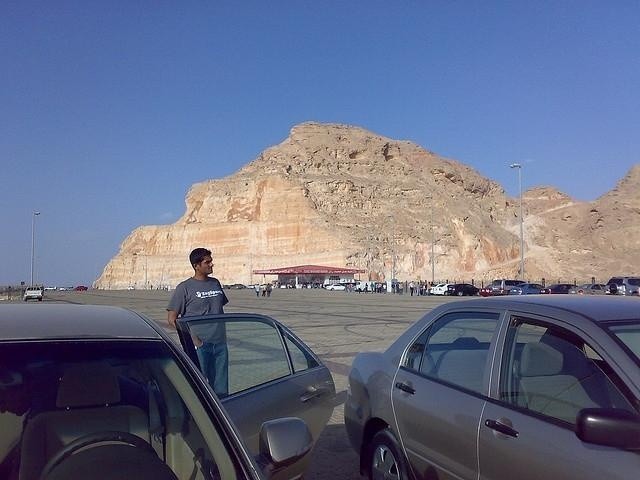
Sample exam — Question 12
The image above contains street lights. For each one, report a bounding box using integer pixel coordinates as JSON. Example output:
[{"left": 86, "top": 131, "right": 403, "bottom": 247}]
[
  {"left": 508, "top": 161, "right": 526, "bottom": 281},
  {"left": 29, "top": 209, "right": 41, "bottom": 286}
]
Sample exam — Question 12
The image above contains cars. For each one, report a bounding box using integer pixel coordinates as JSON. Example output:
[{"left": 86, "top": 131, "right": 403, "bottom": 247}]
[
  {"left": 342, "top": 292, "right": 640, "bottom": 480},
  {"left": 0, "top": 302, "right": 338, "bottom": 478}
]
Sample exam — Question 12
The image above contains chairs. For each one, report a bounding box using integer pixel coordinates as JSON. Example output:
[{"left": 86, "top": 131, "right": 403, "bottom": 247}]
[
  {"left": 412, "top": 340, "right": 606, "bottom": 428},
  {"left": 16, "top": 362, "right": 152, "bottom": 480}
]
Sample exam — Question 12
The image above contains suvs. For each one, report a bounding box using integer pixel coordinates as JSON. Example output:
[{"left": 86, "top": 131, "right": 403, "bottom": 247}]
[{"left": 23, "top": 286, "right": 43, "bottom": 302}]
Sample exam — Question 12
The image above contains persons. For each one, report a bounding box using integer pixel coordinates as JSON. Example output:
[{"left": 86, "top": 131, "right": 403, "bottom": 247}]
[
  {"left": 344, "top": 280, "right": 436, "bottom": 296},
  {"left": 253, "top": 283, "right": 273, "bottom": 297},
  {"left": 166, "top": 247, "right": 230, "bottom": 397}
]
